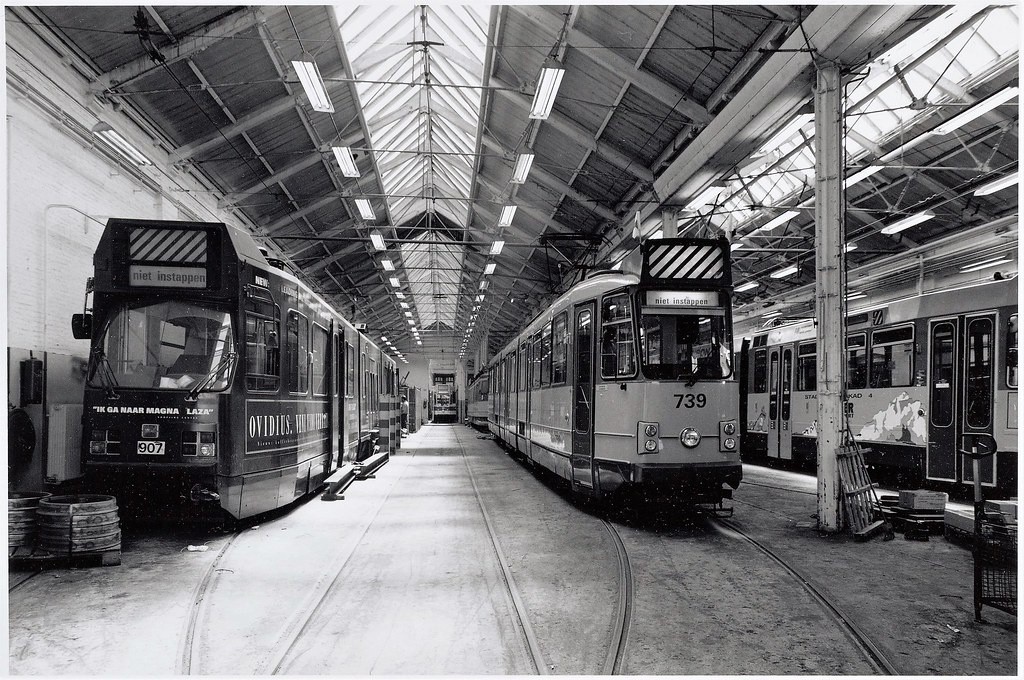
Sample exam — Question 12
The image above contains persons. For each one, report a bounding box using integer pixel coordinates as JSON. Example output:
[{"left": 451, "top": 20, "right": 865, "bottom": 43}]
[{"left": 401, "top": 394, "right": 409, "bottom": 429}]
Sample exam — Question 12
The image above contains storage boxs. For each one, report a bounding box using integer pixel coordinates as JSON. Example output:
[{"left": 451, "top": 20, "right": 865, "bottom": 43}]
[
  {"left": 984, "top": 497, "right": 1017, "bottom": 535},
  {"left": 899, "top": 489, "right": 947, "bottom": 510}
]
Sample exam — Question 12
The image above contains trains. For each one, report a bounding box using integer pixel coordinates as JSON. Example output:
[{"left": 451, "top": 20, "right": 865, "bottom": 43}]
[
  {"left": 734, "top": 273, "right": 1018, "bottom": 500},
  {"left": 72, "top": 218, "right": 403, "bottom": 522},
  {"left": 463, "top": 204, "right": 745, "bottom": 535}
]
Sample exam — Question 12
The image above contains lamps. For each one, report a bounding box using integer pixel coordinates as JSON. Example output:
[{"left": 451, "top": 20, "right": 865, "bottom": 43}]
[
  {"left": 478, "top": 276, "right": 491, "bottom": 290},
  {"left": 332, "top": 138, "right": 360, "bottom": 177},
  {"left": 290, "top": 50, "right": 335, "bottom": 113},
  {"left": 498, "top": 199, "right": 518, "bottom": 228},
  {"left": 381, "top": 256, "right": 395, "bottom": 272},
  {"left": 489, "top": 234, "right": 506, "bottom": 255},
  {"left": 483, "top": 259, "right": 497, "bottom": 275},
  {"left": 510, "top": 146, "right": 535, "bottom": 184},
  {"left": 370, "top": 229, "right": 386, "bottom": 251},
  {"left": 355, "top": 192, "right": 376, "bottom": 220},
  {"left": 730, "top": 77, "right": 1019, "bottom": 292},
  {"left": 389, "top": 274, "right": 400, "bottom": 287},
  {"left": 528, "top": 60, "right": 566, "bottom": 120}
]
